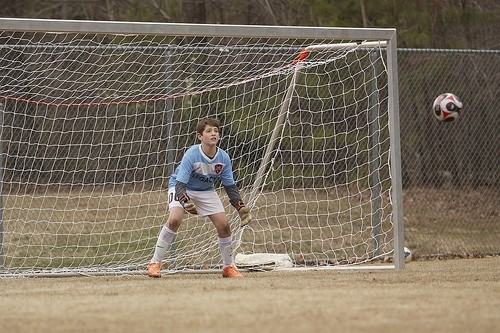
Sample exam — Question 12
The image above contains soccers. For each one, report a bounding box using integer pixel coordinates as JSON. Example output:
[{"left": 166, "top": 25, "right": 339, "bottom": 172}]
[{"left": 434, "top": 93, "right": 464, "bottom": 121}]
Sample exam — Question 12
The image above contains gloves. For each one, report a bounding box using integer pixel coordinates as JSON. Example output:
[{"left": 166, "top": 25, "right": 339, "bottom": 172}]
[
  {"left": 235, "top": 201, "right": 252, "bottom": 227},
  {"left": 180, "top": 194, "right": 199, "bottom": 215}
]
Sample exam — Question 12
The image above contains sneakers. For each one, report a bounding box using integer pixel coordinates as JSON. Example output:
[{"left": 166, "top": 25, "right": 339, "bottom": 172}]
[
  {"left": 223, "top": 267, "right": 245, "bottom": 278},
  {"left": 146, "top": 262, "right": 162, "bottom": 278}
]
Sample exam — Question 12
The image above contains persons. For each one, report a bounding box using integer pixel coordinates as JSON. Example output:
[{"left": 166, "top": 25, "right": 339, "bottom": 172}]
[{"left": 147, "top": 117, "right": 252, "bottom": 281}]
